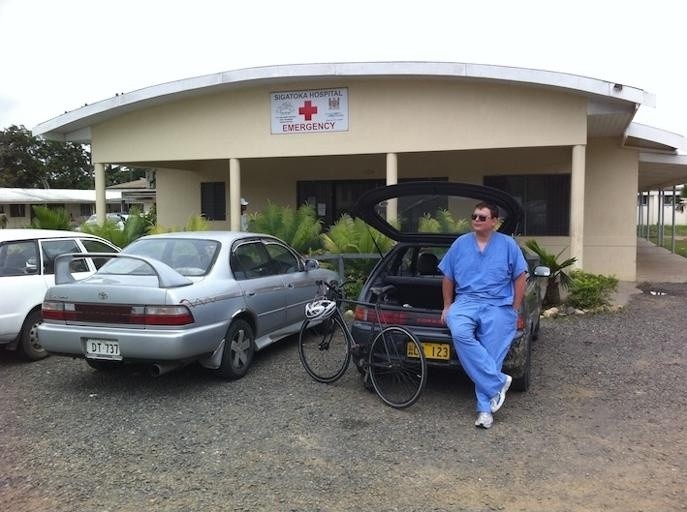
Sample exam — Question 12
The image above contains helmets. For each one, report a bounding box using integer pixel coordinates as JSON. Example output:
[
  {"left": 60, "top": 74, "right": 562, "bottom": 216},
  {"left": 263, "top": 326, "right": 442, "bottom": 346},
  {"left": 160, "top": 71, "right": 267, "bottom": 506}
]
[{"left": 304, "top": 299, "right": 336, "bottom": 321}]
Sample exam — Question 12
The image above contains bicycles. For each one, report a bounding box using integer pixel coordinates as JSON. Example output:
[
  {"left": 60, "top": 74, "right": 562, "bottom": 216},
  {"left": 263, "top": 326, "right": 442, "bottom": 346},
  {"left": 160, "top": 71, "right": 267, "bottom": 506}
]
[{"left": 300, "top": 281, "right": 429, "bottom": 409}]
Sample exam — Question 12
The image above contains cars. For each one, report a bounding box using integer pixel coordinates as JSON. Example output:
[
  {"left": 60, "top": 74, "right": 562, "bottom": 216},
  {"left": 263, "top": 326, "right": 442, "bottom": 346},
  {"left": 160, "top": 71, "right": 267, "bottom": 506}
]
[
  {"left": 353, "top": 181, "right": 553, "bottom": 390},
  {"left": 1, "top": 228, "right": 124, "bottom": 361},
  {"left": 25, "top": 213, "right": 146, "bottom": 275},
  {"left": 39, "top": 231, "right": 343, "bottom": 380}
]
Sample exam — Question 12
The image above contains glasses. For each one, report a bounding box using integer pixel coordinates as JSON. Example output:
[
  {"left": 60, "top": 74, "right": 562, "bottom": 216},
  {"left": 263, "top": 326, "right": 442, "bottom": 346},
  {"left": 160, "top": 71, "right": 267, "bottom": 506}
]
[{"left": 472, "top": 214, "right": 490, "bottom": 222}]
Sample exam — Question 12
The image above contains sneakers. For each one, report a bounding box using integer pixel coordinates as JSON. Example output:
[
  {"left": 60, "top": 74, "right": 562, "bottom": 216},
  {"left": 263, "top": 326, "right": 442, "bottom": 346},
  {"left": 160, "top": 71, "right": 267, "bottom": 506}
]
[{"left": 474, "top": 374, "right": 512, "bottom": 429}]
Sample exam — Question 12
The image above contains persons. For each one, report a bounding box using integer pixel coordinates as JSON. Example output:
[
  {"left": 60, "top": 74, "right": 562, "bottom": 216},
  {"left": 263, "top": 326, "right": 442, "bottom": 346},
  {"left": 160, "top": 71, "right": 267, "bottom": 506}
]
[
  {"left": 240, "top": 197, "right": 251, "bottom": 232},
  {"left": 434, "top": 199, "right": 529, "bottom": 431}
]
